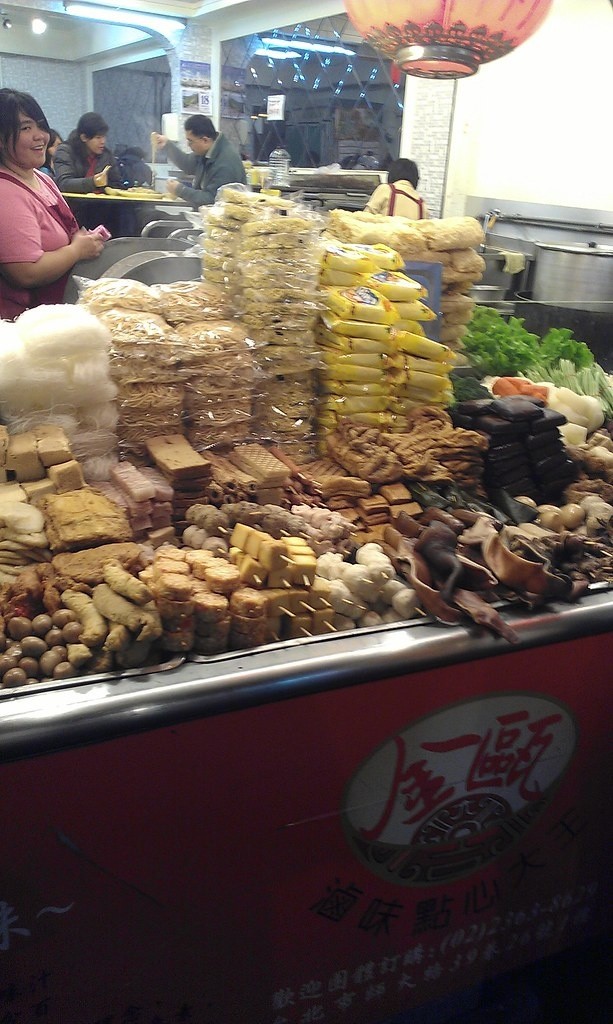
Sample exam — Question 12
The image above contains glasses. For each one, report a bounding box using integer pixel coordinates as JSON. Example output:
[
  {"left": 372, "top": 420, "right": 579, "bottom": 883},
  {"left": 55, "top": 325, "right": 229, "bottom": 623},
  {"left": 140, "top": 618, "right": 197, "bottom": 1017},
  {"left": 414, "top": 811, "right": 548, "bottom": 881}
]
[{"left": 186, "top": 136, "right": 203, "bottom": 146}]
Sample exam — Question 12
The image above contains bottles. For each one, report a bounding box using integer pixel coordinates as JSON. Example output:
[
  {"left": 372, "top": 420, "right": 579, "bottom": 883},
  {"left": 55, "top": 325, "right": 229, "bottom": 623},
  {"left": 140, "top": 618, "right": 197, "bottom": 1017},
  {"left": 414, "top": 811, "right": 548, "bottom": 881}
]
[{"left": 269, "top": 145, "right": 291, "bottom": 188}]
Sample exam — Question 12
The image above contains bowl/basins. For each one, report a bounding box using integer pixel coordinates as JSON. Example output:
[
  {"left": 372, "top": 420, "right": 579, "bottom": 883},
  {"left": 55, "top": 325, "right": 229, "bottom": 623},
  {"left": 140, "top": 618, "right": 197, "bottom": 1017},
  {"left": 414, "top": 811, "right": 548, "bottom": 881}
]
[{"left": 465, "top": 285, "right": 509, "bottom": 300}]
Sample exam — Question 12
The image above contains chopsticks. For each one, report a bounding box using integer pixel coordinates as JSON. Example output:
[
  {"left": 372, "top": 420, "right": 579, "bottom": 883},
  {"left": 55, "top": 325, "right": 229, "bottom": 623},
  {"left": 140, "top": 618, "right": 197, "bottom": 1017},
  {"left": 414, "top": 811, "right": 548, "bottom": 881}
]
[{"left": 103, "top": 165, "right": 110, "bottom": 174}]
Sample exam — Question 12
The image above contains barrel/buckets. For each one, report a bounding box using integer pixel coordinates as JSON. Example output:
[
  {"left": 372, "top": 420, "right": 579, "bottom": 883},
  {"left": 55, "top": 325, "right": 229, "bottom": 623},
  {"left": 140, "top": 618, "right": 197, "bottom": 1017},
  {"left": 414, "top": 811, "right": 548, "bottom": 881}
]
[{"left": 514, "top": 290, "right": 613, "bottom": 376}]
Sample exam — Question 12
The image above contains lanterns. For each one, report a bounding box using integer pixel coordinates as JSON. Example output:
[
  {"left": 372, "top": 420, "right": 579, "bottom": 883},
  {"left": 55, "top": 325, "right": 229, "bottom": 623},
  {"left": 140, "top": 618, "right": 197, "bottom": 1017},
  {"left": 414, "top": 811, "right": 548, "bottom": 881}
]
[{"left": 345, "top": 0, "right": 555, "bottom": 80}]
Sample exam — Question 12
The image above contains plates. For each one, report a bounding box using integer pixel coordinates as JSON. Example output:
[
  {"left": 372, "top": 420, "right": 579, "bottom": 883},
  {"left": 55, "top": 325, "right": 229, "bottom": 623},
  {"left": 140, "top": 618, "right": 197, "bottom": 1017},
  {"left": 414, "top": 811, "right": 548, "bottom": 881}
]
[{"left": 118, "top": 191, "right": 165, "bottom": 200}]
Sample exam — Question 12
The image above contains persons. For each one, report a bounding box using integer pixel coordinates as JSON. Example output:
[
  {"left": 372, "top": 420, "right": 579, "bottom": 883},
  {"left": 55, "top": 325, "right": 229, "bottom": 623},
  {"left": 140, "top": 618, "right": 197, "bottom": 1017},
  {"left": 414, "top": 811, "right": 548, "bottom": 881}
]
[
  {"left": 40, "top": 112, "right": 152, "bottom": 238},
  {"left": 362, "top": 158, "right": 427, "bottom": 220},
  {"left": 137, "top": 115, "right": 247, "bottom": 238},
  {"left": 0, "top": 87, "right": 105, "bottom": 321}
]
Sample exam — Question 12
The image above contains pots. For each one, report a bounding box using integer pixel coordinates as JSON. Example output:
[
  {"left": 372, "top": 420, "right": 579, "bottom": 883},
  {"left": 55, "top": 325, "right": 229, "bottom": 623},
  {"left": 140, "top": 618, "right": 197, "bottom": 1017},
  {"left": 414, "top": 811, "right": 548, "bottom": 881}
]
[{"left": 532, "top": 242, "right": 613, "bottom": 312}]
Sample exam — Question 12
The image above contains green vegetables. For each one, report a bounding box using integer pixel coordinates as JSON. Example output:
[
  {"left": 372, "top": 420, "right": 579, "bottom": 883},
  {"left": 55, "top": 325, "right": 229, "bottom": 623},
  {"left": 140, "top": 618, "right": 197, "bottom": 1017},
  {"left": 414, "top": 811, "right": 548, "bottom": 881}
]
[
  {"left": 512, "top": 360, "right": 613, "bottom": 418},
  {"left": 459, "top": 303, "right": 594, "bottom": 377}
]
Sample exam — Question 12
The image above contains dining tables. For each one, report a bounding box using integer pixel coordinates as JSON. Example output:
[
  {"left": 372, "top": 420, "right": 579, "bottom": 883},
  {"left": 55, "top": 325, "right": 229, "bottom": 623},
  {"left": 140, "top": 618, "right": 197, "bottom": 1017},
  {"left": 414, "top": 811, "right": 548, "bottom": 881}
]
[{"left": 58, "top": 191, "right": 199, "bottom": 237}]
